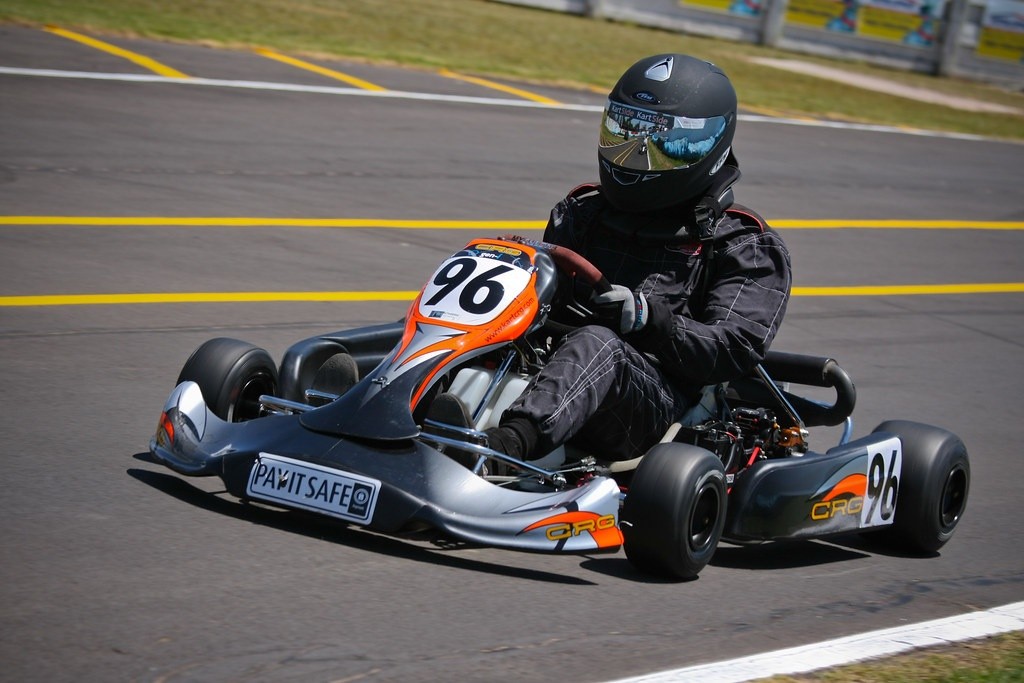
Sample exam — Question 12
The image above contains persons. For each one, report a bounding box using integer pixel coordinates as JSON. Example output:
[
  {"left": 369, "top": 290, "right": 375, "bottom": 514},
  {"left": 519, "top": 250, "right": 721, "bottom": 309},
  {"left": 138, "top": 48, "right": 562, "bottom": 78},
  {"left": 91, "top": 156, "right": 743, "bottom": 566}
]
[{"left": 307, "top": 51, "right": 792, "bottom": 487}]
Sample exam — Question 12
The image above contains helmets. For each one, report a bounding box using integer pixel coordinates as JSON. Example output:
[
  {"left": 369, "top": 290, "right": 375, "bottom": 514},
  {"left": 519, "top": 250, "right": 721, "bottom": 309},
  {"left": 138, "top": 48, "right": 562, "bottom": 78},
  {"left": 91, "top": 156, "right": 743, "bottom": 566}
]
[{"left": 598, "top": 54, "right": 737, "bottom": 220}]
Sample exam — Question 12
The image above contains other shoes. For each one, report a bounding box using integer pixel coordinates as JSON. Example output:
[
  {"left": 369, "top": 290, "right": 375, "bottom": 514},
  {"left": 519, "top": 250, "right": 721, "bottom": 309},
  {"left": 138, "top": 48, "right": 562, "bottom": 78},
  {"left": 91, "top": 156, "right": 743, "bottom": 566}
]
[
  {"left": 423, "top": 394, "right": 523, "bottom": 484},
  {"left": 308, "top": 353, "right": 364, "bottom": 408}
]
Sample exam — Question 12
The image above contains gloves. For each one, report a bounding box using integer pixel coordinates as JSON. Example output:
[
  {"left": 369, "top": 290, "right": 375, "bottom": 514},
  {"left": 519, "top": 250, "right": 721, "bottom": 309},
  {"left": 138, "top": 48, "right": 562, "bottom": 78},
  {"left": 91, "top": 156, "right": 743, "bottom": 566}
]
[{"left": 588, "top": 284, "right": 649, "bottom": 337}]
